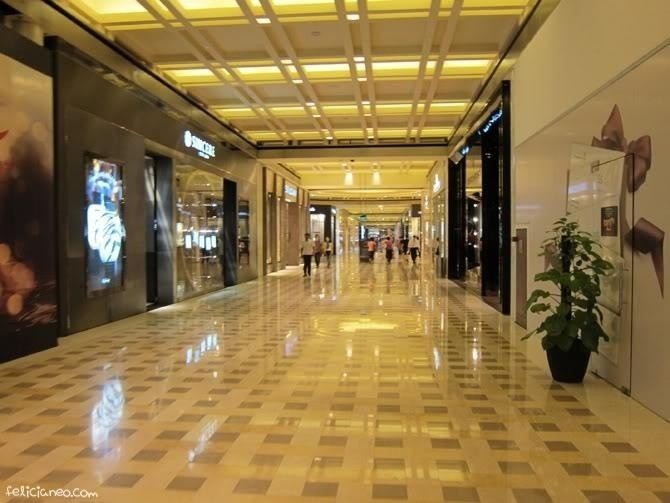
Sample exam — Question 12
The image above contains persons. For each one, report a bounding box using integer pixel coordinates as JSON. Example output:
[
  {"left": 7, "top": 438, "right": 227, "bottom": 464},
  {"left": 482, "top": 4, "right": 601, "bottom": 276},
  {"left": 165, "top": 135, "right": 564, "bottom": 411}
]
[
  {"left": 367, "top": 238, "right": 376, "bottom": 264},
  {"left": 321, "top": 237, "right": 333, "bottom": 268},
  {"left": 396, "top": 235, "right": 420, "bottom": 264},
  {"left": 384, "top": 237, "right": 394, "bottom": 264},
  {"left": 313, "top": 234, "right": 322, "bottom": 268},
  {"left": 301, "top": 233, "right": 315, "bottom": 277}
]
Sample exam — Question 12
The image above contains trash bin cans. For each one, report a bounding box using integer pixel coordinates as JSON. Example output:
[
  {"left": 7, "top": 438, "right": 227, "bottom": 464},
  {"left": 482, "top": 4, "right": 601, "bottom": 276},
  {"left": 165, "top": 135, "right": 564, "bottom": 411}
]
[{"left": 436, "top": 257, "right": 446, "bottom": 278}]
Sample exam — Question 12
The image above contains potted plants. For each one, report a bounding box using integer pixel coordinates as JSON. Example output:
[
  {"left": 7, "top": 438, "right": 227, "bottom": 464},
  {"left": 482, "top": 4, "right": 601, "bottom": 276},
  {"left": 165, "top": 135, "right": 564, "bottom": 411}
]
[{"left": 520, "top": 211, "right": 618, "bottom": 384}]
